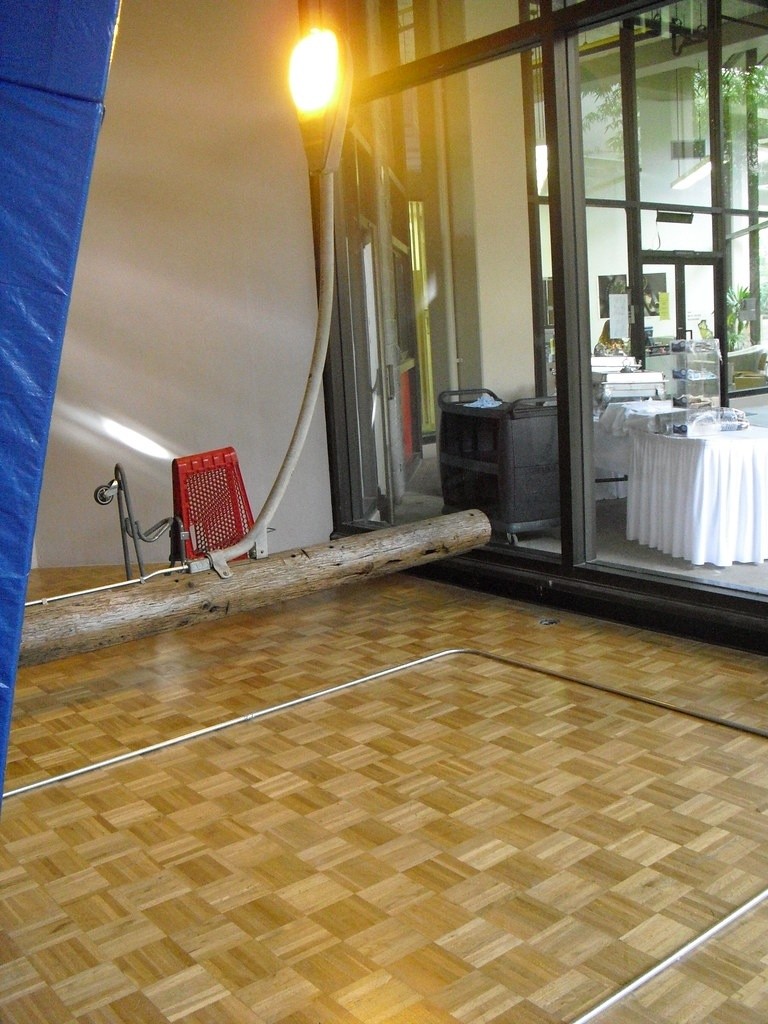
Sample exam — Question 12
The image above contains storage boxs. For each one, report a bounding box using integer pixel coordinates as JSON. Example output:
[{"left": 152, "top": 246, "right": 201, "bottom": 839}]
[{"left": 592, "top": 354, "right": 669, "bottom": 406}]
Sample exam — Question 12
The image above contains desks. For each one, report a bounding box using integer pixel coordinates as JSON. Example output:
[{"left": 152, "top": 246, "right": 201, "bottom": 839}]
[
  {"left": 592, "top": 400, "right": 768, "bottom": 566},
  {"left": 646, "top": 338, "right": 721, "bottom": 403}
]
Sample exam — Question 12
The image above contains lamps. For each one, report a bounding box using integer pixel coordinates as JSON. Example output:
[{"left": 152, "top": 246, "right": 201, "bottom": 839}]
[{"left": 181, "top": 14, "right": 355, "bottom": 574}]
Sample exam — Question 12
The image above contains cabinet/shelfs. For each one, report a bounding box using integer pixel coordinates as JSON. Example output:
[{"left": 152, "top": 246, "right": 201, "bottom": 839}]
[{"left": 735, "top": 373, "right": 765, "bottom": 390}]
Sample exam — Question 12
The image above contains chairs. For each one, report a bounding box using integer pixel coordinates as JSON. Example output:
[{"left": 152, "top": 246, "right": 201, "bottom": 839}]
[{"left": 727, "top": 345, "right": 764, "bottom": 371}]
